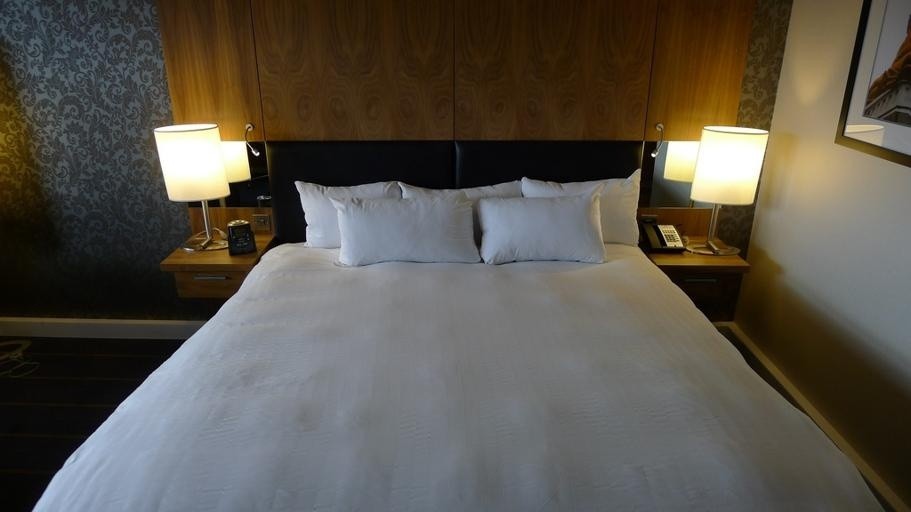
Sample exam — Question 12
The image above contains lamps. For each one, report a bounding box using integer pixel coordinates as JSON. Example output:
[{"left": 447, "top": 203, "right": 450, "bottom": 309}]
[
  {"left": 222, "top": 142, "right": 252, "bottom": 183},
  {"left": 662, "top": 141, "right": 699, "bottom": 208},
  {"left": 683, "top": 126, "right": 769, "bottom": 257},
  {"left": 154, "top": 123, "right": 231, "bottom": 251}
]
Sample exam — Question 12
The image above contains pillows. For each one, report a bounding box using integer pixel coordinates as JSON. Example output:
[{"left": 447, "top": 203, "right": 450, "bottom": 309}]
[
  {"left": 472, "top": 183, "right": 608, "bottom": 265},
  {"left": 328, "top": 194, "right": 483, "bottom": 264},
  {"left": 397, "top": 181, "right": 522, "bottom": 204},
  {"left": 291, "top": 179, "right": 401, "bottom": 249},
  {"left": 520, "top": 169, "right": 642, "bottom": 247}
]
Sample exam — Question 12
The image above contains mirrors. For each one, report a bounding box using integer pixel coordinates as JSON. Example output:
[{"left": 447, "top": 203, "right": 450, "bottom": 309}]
[
  {"left": 640, "top": 141, "right": 722, "bottom": 211},
  {"left": 184, "top": 140, "right": 272, "bottom": 207}
]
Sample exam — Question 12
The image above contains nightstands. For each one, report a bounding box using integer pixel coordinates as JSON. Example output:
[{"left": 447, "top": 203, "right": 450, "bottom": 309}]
[
  {"left": 159, "top": 233, "right": 274, "bottom": 320},
  {"left": 646, "top": 234, "right": 751, "bottom": 323}
]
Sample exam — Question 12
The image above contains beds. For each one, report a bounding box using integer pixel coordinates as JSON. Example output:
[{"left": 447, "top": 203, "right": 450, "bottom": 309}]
[{"left": 26, "top": 140, "right": 887, "bottom": 511}]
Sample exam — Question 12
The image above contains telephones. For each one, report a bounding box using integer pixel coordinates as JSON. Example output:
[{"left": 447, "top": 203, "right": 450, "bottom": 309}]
[{"left": 640, "top": 214, "right": 687, "bottom": 253}]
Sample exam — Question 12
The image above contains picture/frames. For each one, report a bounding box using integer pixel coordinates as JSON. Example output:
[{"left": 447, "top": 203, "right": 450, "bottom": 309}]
[{"left": 832, "top": 0, "right": 910, "bottom": 168}]
